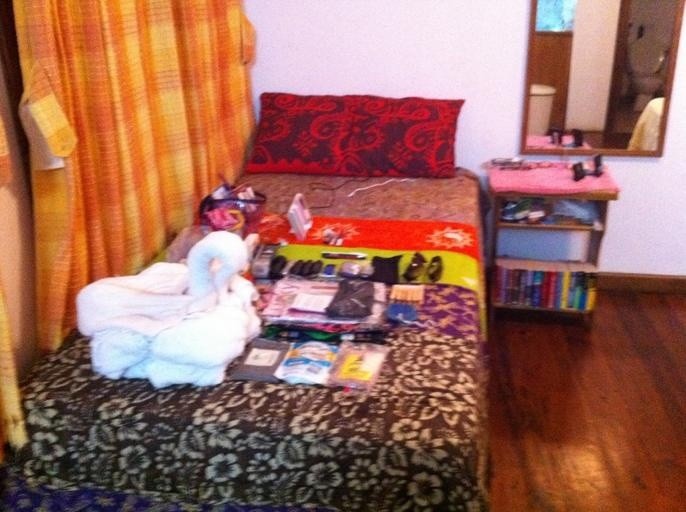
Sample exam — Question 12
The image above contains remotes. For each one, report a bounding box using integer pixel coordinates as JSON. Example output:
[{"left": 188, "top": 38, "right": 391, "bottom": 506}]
[{"left": 491, "top": 157, "right": 523, "bottom": 164}]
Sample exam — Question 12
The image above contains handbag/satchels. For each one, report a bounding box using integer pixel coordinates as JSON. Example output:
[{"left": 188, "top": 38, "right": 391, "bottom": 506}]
[{"left": 200, "top": 183, "right": 266, "bottom": 233}]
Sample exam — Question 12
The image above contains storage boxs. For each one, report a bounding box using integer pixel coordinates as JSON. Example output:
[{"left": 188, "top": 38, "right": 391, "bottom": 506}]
[
  {"left": 527, "top": 84, "right": 556, "bottom": 137},
  {"left": 497, "top": 228, "right": 591, "bottom": 262}
]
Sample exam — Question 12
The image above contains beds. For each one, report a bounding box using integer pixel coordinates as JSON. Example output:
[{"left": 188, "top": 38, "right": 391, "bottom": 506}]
[{"left": 0, "top": 168, "right": 491, "bottom": 511}]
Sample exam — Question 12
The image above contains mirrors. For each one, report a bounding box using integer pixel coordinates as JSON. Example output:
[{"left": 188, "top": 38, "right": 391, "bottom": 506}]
[{"left": 520, "top": 0, "right": 686, "bottom": 158}]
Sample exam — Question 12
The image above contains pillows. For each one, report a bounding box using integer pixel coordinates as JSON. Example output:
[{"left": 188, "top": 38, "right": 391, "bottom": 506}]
[{"left": 244, "top": 92, "right": 466, "bottom": 179}]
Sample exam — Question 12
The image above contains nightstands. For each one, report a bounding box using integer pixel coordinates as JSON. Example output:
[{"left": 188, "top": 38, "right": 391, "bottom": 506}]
[{"left": 483, "top": 161, "right": 620, "bottom": 322}]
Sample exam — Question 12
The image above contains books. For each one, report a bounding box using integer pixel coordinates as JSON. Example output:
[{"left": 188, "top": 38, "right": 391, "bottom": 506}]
[{"left": 493, "top": 256, "right": 597, "bottom": 314}]
